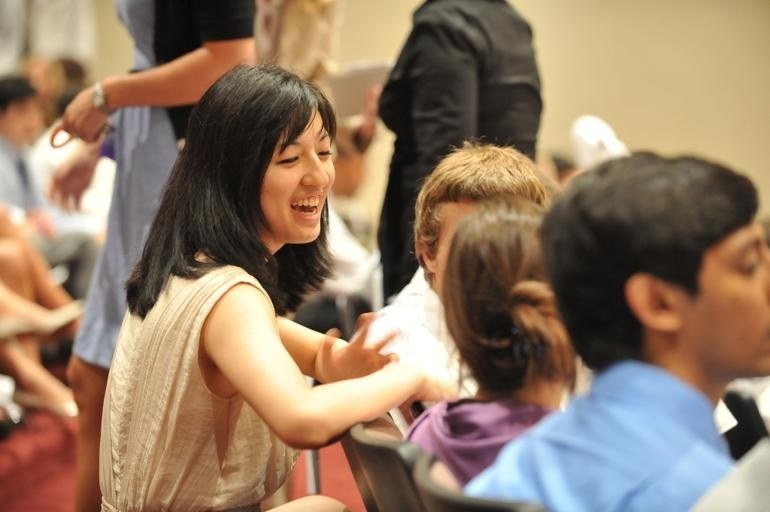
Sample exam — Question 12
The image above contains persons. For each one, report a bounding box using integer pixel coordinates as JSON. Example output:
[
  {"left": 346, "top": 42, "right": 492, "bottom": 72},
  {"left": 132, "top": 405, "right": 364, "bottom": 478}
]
[{"left": 0, "top": 0, "right": 542, "bottom": 512}]
